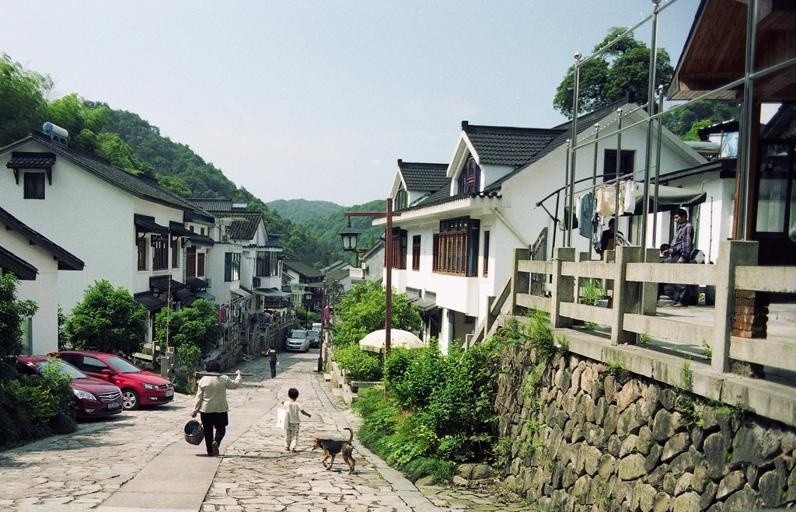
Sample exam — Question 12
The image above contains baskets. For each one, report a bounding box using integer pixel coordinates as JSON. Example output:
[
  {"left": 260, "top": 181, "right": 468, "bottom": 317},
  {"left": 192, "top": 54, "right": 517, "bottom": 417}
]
[{"left": 183, "top": 419, "right": 204, "bottom": 446}]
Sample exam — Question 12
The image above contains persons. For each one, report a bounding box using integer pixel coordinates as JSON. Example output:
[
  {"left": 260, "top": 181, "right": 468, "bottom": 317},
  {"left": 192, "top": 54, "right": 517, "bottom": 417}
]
[
  {"left": 601, "top": 218, "right": 623, "bottom": 253},
  {"left": 268, "top": 348, "right": 277, "bottom": 379},
  {"left": 661, "top": 209, "right": 705, "bottom": 307},
  {"left": 281, "top": 388, "right": 311, "bottom": 453},
  {"left": 192, "top": 360, "right": 242, "bottom": 456}
]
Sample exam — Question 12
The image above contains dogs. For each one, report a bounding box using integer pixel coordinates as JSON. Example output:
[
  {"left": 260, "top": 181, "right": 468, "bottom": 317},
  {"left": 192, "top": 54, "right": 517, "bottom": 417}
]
[{"left": 312, "top": 428, "right": 355, "bottom": 475}]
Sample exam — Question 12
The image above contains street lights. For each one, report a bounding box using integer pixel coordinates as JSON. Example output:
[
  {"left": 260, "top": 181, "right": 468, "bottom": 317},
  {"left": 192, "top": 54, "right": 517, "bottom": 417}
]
[
  {"left": 338, "top": 198, "right": 401, "bottom": 359},
  {"left": 304, "top": 282, "right": 326, "bottom": 372}
]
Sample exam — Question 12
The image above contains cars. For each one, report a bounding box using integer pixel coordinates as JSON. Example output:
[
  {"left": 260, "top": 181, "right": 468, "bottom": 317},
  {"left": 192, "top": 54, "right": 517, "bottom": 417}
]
[
  {"left": 14, "top": 351, "right": 174, "bottom": 419},
  {"left": 286, "top": 323, "right": 323, "bottom": 352}
]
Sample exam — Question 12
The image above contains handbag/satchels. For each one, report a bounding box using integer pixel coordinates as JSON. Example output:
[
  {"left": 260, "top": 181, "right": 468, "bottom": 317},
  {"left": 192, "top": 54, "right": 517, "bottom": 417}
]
[{"left": 277, "top": 402, "right": 289, "bottom": 430}]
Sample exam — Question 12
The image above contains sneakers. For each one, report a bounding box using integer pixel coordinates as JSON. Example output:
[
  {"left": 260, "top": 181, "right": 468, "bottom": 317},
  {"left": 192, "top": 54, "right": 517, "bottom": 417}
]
[
  {"left": 284, "top": 446, "right": 296, "bottom": 452},
  {"left": 212, "top": 441, "right": 219, "bottom": 455}
]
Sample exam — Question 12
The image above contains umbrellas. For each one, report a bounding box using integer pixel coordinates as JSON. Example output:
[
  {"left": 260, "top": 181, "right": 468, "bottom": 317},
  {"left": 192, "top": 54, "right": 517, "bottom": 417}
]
[{"left": 359, "top": 329, "right": 425, "bottom": 354}]
